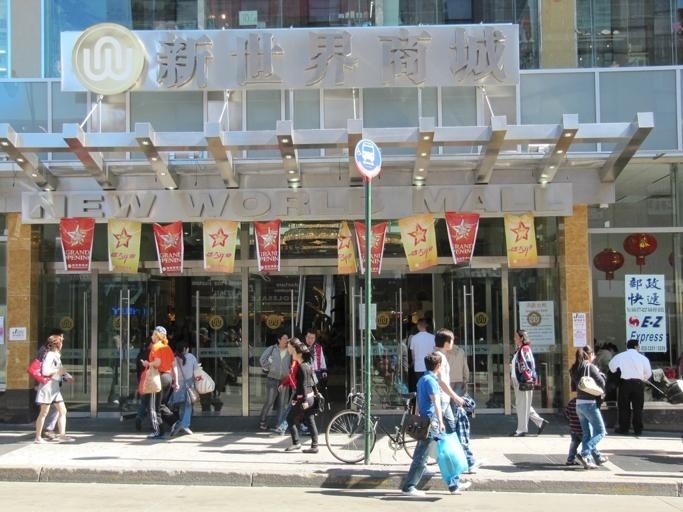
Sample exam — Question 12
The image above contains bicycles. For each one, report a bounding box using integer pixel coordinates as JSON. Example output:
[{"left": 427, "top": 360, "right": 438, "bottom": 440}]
[{"left": 324, "top": 393, "right": 442, "bottom": 466}]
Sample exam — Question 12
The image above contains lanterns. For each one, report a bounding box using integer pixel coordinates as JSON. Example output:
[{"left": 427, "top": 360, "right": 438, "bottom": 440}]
[
  {"left": 624, "top": 233, "right": 658, "bottom": 267},
  {"left": 593, "top": 247, "right": 623, "bottom": 282}
]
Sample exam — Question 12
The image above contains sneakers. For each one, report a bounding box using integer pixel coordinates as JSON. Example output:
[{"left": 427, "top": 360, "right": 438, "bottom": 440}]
[
  {"left": 285, "top": 443, "right": 318, "bottom": 452},
  {"left": 35, "top": 438, "right": 47, "bottom": 444},
  {"left": 42, "top": 426, "right": 55, "bottom": 439},
  {"left": 565, "top": 454, "right": 610, "bottom": 469},
  {"left": 451, "top": 482, "right": 472, "bottom": 494},
  {"left": 612, "top": 427, "right": 644, "bottom": 436},
  {"left": 402, "top": 487, "right": 424, "bottom": 496},
  {"left": 59, "top": 437, "right": 76, "bottom": 443},
  {"left": 257, "top": 418, "right": 322, "bottom": 435},
  {"left": 144, "top": 420, "right": 194, "bottom": 439}
]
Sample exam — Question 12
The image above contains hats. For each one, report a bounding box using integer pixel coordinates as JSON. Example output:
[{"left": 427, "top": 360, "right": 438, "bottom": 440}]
[{"left": 155, "top": 326, "right": 167, "bottom": 335}]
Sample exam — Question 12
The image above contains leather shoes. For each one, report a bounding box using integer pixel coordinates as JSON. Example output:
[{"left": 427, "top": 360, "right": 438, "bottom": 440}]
[
  {"left": 509, "top": 432, "right": 526, "bottom": 436},
  {"left": 536, "top": 420, "right": 549, "bottom": 434}
]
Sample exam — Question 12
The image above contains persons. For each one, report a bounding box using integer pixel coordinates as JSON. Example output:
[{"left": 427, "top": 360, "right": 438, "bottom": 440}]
[
  {"left": 193, "top": 325, "right": 241, "bottom": 414},
  {"left": 259, "top": 330, "right": 329, "bottom": 453},
  {"left": 432, "top": 330, "right": 483, "bottom": 474},
  {"left": 408, "top": 318, "right": 436, "bottom": 407},
  {"left": 608, "top": 339, "right": 653, "bottom": 435},
  {"left": 108, "top": 327, "right": 129, "bottom": 407},
  {"left": 33, "top": 335, "right": 74, "bottom": 443},
  {"left": 140, "top": 324, "right": 184, "bottom": 438},
  {"left": 38, "top": 328, "right": 72, "bottom": 439},
  {"left": 598, "top": 343, "right": 617, "bottom": 378},
  {"left": 369, "top": 326, "right": 411, "bottom": 409},
  {"left": 170, "top": 339, "right": 203, "bottom": 435},
  {"left": 508, "top": 329, "right": 549, "bottom": 436},
  {"left": 567, "top": 345, "right": 608, "bottom": 469},
  {"left": 401, "top": 353, "right": 472, "bottom": 496},
  {"left": 566, "top": 396, "right": 608, "bottom": 467},
  {"left": 135, "top": 341, "right": 154, "bottom": 429}
]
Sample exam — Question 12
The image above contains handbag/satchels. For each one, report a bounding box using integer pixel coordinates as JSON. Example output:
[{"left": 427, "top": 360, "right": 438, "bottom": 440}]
[
  {"left": 307, "top": 389, "right": 325, "bottom": 417},
  {"left": 27, "top": 358, "right": 51, "bottom": 384},
  {"left": 187, "top": 387, "right": 200, "bottom": 403},
  {"left": 407, "top": 415, "right": 431, "bottom": 439},
  {"left": 578, "top": 367, "right": 604, "bottom": 396},
  {"left": 519, "top": 382, "right": 532, "bottom": 391}
]
